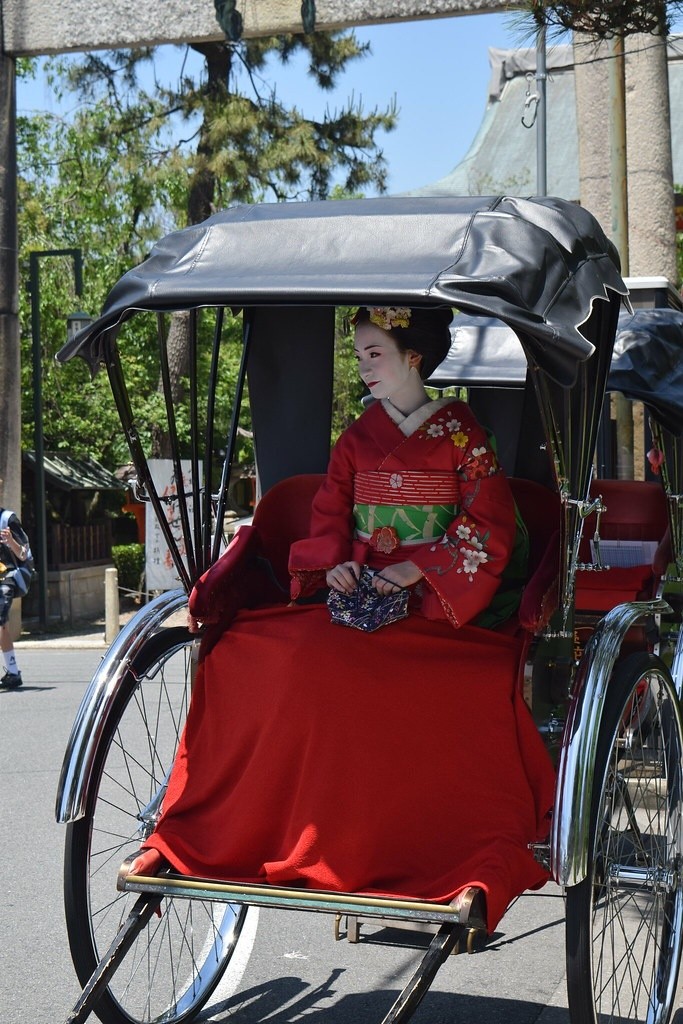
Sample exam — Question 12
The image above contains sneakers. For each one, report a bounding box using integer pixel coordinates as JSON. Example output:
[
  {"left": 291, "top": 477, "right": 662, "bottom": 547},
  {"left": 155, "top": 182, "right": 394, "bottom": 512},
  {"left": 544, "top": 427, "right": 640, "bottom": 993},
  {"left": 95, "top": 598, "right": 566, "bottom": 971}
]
[{"left": 0, "top": 671, "right": 22, "bottom": 689}]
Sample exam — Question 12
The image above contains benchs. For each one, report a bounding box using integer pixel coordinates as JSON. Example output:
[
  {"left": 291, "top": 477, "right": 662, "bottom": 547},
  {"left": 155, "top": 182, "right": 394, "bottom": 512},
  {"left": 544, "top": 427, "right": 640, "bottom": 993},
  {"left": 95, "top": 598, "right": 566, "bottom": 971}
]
[
  {"left": 188, "top": 473, "right": 566, "bottom": 698},
  {"left": 574, "top": 480, "right": 672, "bottom": 615}
]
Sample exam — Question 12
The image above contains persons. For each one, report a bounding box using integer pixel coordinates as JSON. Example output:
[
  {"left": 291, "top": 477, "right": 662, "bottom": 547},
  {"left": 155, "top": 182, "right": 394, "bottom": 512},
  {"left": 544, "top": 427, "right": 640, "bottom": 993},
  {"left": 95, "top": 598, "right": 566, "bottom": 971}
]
[
  {"left": 0, "top": 479, "right": 35, "bottom": 691},
  {"left": 203, "top": 301, "right": 530, "bottom": 886}
]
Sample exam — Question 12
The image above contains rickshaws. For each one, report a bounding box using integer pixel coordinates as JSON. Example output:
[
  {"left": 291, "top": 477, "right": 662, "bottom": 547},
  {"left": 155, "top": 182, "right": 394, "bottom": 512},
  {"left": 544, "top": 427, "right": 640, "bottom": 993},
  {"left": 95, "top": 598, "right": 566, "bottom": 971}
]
[{"left": 41, "top": 186, "right": 682, "bottom": 1024}]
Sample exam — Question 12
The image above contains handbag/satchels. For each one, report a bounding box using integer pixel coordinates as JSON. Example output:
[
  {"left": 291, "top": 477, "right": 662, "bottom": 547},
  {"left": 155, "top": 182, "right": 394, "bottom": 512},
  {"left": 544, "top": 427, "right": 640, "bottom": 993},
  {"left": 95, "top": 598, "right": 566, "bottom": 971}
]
[
  {"left": 0, "top": 509, "right": 37, "bottom": 598},
  {"left": 326, "top": 566, "right": 410, "bottom": 631}
]
